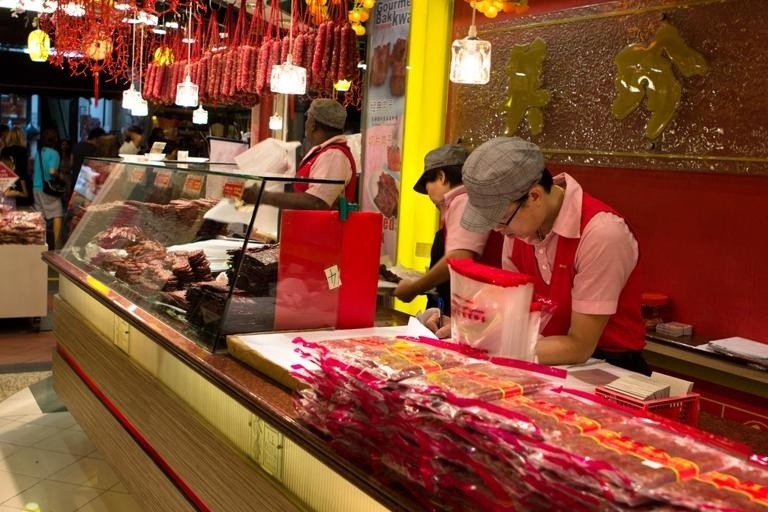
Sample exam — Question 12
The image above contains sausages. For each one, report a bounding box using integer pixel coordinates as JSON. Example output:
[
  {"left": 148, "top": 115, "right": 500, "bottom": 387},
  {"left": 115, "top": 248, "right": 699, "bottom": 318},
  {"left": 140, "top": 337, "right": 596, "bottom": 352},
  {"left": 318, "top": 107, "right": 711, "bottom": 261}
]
[
  {"left": 290, "top": 336, "right": 768, "bottom": 512},
  {"left": 143, "top": 19, "right": 362, "bottom": 109}
]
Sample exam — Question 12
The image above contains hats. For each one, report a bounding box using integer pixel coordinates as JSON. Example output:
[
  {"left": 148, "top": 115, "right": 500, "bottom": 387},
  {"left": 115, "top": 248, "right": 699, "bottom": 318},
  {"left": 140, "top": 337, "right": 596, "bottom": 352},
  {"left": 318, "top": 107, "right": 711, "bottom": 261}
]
[
  {"left": 304, "top": 98, "right": 346, "bottom": 129},
  {"left": 460, "top": 135, "right": 544, "bottom": 234},
  {"left": 412, "top": 145, "right": 466, "bottom": 194}
]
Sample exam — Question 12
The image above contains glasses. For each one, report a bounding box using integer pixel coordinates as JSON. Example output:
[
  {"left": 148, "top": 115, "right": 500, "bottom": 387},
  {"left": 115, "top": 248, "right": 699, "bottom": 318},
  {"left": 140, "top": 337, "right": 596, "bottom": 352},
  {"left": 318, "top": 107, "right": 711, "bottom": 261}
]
[{"left": 498, "top": 197, "right": 523, "bottom": 226}]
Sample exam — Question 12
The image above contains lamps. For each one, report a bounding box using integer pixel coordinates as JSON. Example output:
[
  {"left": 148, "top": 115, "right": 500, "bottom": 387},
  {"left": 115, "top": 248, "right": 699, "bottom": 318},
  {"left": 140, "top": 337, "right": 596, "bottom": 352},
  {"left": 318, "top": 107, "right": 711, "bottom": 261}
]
[
  {"left": 23, "top": 0, "right": 306, "bottom": 133},
  {"left": 449, "top": 0, "right": 493, "bottom": 87}
]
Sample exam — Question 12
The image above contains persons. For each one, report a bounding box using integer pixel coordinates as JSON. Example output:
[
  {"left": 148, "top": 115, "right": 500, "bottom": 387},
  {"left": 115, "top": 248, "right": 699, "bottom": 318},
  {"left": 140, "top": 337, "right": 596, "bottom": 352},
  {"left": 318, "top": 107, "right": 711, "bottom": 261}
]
[
  {"left": 240, "top": 98, "right": 357, "bottom": 210},
  {"left": 388, "top": 144, "right": 504, "bottom": 318},
  {"left": 0, "top": 114, "right": 179, "bottom": 251},
  {"left": 418, "top": 136, "right": 651, "bottom": 376}
]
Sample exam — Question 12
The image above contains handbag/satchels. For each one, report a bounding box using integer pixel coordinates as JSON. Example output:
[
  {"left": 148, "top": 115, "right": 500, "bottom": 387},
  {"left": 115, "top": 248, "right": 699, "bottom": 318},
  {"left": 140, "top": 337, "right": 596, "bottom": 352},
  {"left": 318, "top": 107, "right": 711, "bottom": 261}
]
[{"left": 44, "top": 181, "right": 66, "bottom": 197}]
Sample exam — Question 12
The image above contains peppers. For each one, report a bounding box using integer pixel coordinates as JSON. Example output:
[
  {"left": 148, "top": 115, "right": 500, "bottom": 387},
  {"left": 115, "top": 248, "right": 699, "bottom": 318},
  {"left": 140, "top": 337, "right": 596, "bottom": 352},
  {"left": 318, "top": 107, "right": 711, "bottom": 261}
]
[{"left": 40, "top": 1, "right": 180, "bottom": 85}]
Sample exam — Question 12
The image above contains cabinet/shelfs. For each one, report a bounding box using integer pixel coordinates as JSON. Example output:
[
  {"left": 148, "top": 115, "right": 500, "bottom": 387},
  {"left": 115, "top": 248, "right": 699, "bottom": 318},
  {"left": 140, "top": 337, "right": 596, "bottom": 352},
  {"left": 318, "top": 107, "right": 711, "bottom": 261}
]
[{"left": 56, "top": 152, "right": 386, "bottom": 352}]
